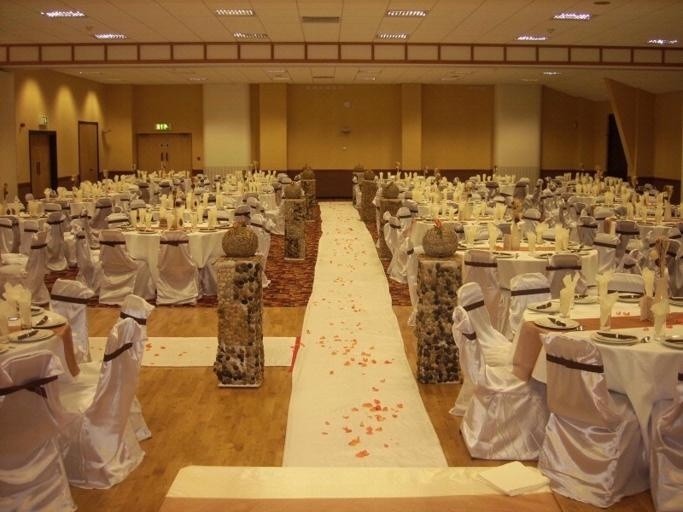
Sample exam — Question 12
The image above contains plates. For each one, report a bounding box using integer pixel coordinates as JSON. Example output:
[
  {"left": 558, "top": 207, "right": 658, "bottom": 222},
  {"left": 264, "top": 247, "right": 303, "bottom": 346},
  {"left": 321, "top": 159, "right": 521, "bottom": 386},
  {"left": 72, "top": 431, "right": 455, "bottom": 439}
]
[
  {"left": 492, "top": 240, "right": 593, "bottom": 259},
  {"left": 527, "top": 291, "right": 683, "bottom": 350},
  {"left": 0, "top": 306, "right": 66, "bottom": 355},
  {"left": 198, "top": 222, "right": 234, "bottom": 233},
  {"left": 122, "top": 224, "right": 158, "bottom": 234}
]
